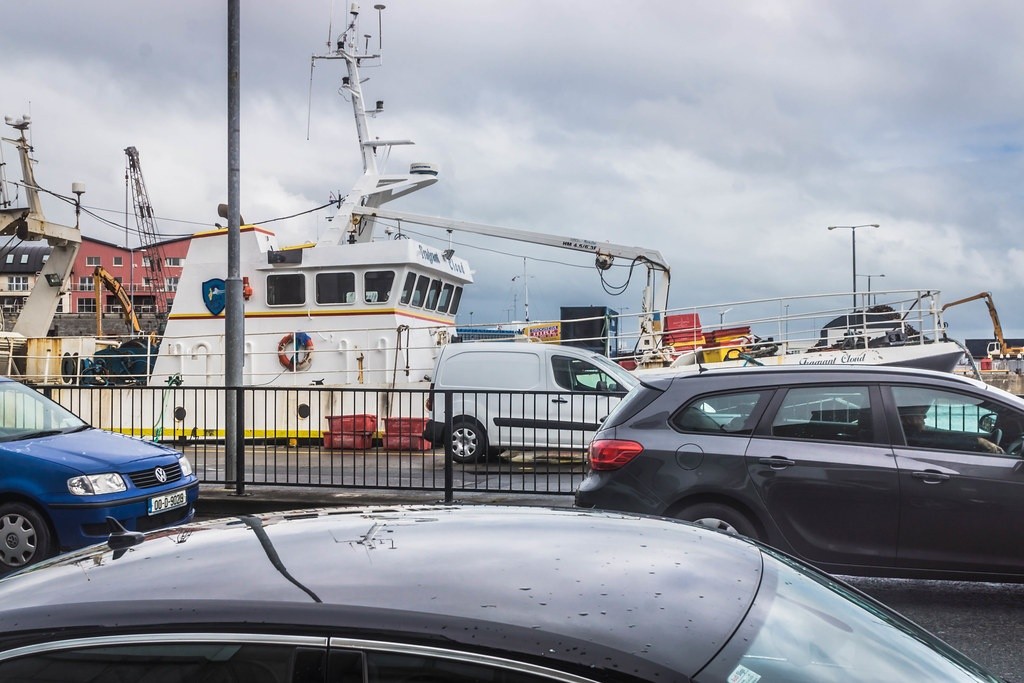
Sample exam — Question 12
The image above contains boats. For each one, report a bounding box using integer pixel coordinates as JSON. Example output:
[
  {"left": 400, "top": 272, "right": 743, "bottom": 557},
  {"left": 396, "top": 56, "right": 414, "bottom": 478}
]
[{"left": 0, "top": 0, "right": 966, "bottom": 450}]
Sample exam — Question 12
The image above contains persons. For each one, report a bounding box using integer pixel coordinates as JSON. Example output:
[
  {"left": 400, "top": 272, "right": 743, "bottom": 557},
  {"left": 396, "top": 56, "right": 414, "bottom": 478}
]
[{"left": 897, "top": 393, "right": 1006, "bottom": 456}]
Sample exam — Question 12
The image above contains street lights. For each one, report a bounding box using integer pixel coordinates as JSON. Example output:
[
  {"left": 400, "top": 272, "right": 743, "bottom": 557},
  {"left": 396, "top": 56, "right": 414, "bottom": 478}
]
[
  {"left": 615, "top": 306, "right": 629, "bottom": 354},
  {"left": 784, "top": 305, "right": 791, "bottom": 352},
  {"left": 828, "top": 224, "right": 880, "bottom": 352},
  {"left": 718, "top": 312, "right": 724, "bottom": 329},
  {"left": 856, "top": 274, "right": 887, "bottom": 330}
]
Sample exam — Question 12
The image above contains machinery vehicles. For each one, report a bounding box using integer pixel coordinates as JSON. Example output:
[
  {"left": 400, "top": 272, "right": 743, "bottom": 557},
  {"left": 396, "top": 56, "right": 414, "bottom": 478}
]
[
  {"left": 92, "top": 266, "right": 159, "bottom": 348},
  {"left": 940, "top": 289, "right": 1024, "bottom": 362}
]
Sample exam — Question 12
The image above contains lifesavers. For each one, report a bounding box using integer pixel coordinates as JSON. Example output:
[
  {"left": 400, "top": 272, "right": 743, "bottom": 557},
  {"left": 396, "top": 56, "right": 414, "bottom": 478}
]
[{"left": 277, "top": 331, "right": 314, "bottom": 372}]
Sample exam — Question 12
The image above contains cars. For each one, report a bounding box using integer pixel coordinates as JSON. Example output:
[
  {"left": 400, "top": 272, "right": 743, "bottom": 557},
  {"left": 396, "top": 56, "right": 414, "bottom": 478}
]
[
  {"left": 0, "top": 501, "right": 1007, "bottom": 682},
  {"left": 424, "top": 343, "right": 715, "bottom": 464},
  {"left": 574, "top": 343, "right": 1024, "bottom": 584},
  {"left": 0, "top": 377, "right": 198, "bottom": 582}
]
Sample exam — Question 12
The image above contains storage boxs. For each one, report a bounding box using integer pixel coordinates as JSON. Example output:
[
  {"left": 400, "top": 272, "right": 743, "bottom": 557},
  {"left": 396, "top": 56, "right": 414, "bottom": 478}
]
[
  {"left": 664, "top": 313, "right": 752, "bottom": 363},
  {"left": 323, "top": 413, "right": 432, "bottom": 451}
]
